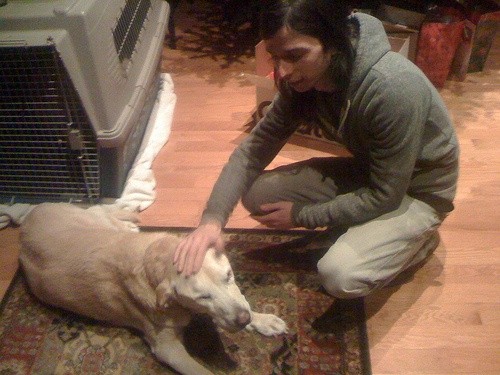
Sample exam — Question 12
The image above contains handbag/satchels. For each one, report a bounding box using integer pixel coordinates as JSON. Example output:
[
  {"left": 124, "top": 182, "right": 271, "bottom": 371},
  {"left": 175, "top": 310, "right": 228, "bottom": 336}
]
[{"left": 416, "top": 9, "right": 500, "bottom": 88}]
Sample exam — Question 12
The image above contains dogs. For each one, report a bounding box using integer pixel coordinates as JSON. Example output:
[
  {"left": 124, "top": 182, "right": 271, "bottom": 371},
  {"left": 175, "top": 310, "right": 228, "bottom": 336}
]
[{"left": 19, "top": 203, "right": 290, "bottom": 375}]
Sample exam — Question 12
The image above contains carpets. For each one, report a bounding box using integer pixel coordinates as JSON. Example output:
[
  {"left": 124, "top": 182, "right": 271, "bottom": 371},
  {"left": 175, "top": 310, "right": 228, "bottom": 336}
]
[{"left": 0, "top": 227, "right": 373, "bottom": 375}]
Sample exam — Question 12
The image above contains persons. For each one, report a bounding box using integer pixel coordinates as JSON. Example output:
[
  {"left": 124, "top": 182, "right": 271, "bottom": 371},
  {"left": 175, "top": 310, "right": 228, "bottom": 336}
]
[{"left": 174, "top": 0, "right": 460, "bottom": 296}]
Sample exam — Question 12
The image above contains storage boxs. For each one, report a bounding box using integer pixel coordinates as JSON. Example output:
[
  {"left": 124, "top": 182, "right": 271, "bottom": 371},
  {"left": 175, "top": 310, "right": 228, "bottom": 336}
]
[{"left": 255, "top": 21, "right": 419, "bottom": 147}]
